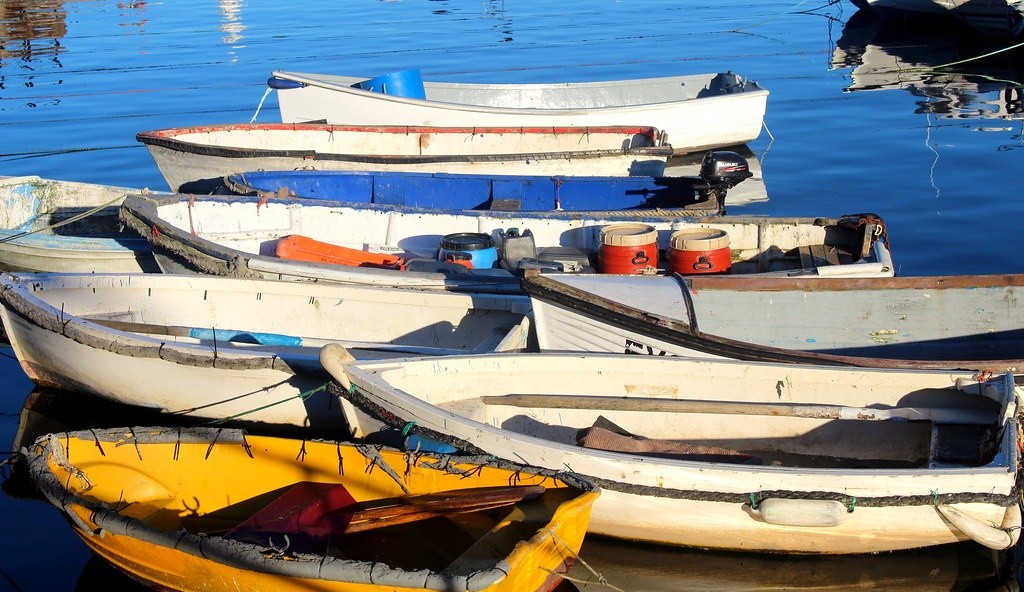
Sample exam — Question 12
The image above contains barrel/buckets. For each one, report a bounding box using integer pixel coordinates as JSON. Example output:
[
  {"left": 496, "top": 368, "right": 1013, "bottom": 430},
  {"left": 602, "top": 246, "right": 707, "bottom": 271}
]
[
  {"left": 668, "top": 228, "right": 732, "bottom": 276},
  {"left": 405, "top": 434, "right": 458, "bottom": 454},
  {"left": 438, "top": 232, "right": 497, "bottom": 270},
  {"left": 359, "top": 68, "right": 426, "bottom": 101},
  {"left": 598, "top": 223, "right": 659, "bottom": 274}
]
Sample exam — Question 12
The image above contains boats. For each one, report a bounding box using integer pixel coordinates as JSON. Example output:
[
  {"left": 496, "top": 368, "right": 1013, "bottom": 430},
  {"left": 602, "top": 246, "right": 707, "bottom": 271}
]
[
  {"left": 114, "top": 187, "right": 898, "bottom": 296},
  {"left": 0, "top": 175, "right": 203, "bottom": 277},
  {"left": 266, "top": 66, "right": 771, "bottom": 153},
  {"left": 834, "top": 14, "right": 1024, "bottom": 203},
  {"left": 500, "top": 228, "right": 1024, "bottom": 387},
  {"left": 0, "top": 271, "right": 541, "bottom": 442},
  {"left": 661, "top": 144, "right": 769, "bottom": 208},
  {"left": 182, "top": 149, "right": 756, "bottom": 222},
  {"left": 316, "top": 341, "right": 1024, "bottom": 558},
  {"left": 13, "top": 425, "right": 602, "bottom": 592},
  {"left": 135, "top": 124, "right": 676, "bottom": 193},
  {"left": 849, "top": 0, "right": 1024, "bottom": 63}
]
